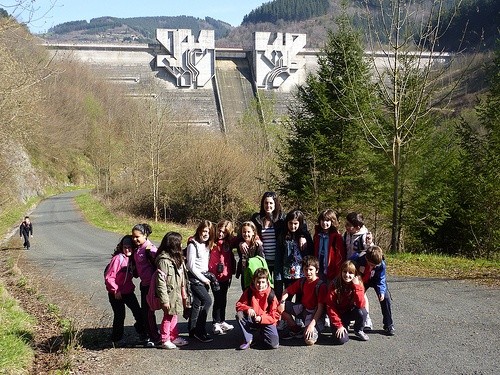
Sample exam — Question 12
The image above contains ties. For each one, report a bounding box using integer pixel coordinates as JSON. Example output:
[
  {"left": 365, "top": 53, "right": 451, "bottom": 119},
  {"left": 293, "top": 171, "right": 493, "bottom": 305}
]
[{"left": 319, "top": 238, "right": 325, "bottom": 272}]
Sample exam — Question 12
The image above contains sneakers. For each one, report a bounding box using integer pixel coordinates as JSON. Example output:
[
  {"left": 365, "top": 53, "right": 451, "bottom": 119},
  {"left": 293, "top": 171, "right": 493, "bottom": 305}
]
[
  {"left": 354, "top": 330, "right": 369, "bottom": 341},
  {"left": 172, "top": 337, "right": 187, "bottom": 346},
  {"left": 159, "top": 341, "right": 176, "bottom": 349},
  {"left": 383, "top": 324, "right": 395, "bottom": 336},
  {"left": 212, "top": 322, "right": 235, "bottom": 335},
  {"left": 363, "top": 327, "right": 371, "bottom": 332}
]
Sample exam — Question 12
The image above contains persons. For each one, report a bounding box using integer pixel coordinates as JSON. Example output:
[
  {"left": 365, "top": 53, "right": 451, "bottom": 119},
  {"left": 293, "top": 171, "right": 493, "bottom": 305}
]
[
  {"left": 104, "top": 191, "right": 395, "bottom": 350},
  {"left": 20, "top": 216, "right": 34, "bottom": 250}
]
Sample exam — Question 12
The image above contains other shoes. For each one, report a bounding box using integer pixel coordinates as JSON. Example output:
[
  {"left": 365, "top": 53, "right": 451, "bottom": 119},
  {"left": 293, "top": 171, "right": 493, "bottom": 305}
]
[
  {"left": 145, "top": 338, "right": 154, "bottom": 347},
  {"left": 191, "top": 336, "right": 214, "bottom": 344},
  {"left": 240, "top": 339, "right": 255, "bottom": 349},
  {"left": 283, "top": 330, "right": 303, "bottom": 339}
]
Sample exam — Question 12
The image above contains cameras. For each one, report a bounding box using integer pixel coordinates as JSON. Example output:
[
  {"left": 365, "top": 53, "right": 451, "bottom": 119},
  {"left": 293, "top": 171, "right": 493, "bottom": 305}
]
[{"left": 217, "top": 263, "right": 224, "bottom": 273}]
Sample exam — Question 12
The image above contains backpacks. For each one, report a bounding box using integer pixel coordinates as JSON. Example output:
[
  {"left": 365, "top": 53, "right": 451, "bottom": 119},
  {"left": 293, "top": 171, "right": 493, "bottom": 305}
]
[
  {"left": 182, "top": 240, "right": 198, "bottom": 265},
  {"left": 299, "top": 273, "right": 328, "bottom": 297},
  {"left": 146, "top": 269, "right": 165, "bottom": 311}
]
